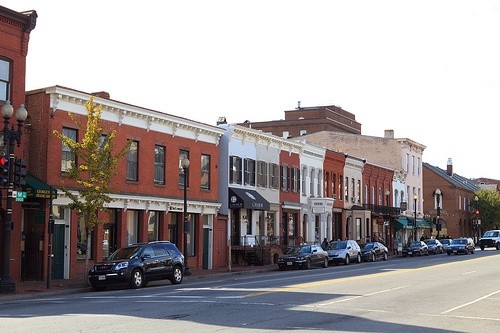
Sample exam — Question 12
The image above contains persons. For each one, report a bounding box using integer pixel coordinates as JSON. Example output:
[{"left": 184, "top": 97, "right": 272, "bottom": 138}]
[
  {"left": 337, "top": 239, "right": 340, "bottom": 242},
  {"left": 344, "top": 237, "right": 348, "bottom": 240},
  {"left": 366, "top": 233, "right": 381, "bottom": 243},
  {"left": 323, "top": 238, "right": 328, "bottom": 247},
  {"left": 408, "top": 235, "right": 413, "bottom": 243},
  {"left": 420, "top": 234, "right": 449, "bottom": 240},
  {"left": 393, "top": 237, "right": 399, "bottom": 255}
]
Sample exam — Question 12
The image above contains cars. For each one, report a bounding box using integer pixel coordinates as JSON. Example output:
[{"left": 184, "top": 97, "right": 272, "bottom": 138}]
[
  {"left": 276, "top": 245, "right": 330, "bottom": 271},
  {"left": 360, "top": 241, "right": 389, "bottom": 262},
  {"left": 402, "top": 241, "right": 429, "bottom": 257},
  {"left": 423, "top": 238, "right": 443, "bottom": 254},
  {"left": 440, "top": 239, "right": 452, "bottom": 250}
]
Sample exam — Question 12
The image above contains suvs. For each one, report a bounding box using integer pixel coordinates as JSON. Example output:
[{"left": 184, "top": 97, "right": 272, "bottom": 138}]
[
  {"left": 324, "top": 240, "right": 363, "bottom": 264},
  {"left": 446, "top": 238, "right": 476, "bottom": 255},
  {"left": 478, "top": 230, "right": 500, "bottom": 250},
  {"left": 89, "top": 240, "right": 185, "bottom": 292}
]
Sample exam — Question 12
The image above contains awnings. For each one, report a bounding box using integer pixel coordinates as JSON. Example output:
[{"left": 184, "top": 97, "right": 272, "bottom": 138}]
[
  {"left": 228, "top": 187, "right": 270, "bottom": 210},
  {"left": 21, "top": 174, "right": 57, "bottom": 198},
  {"left": 395, "top": 219, "right": 415, "bottom": 229},
  {"left": 407, "top": 217, "right": 430, "bottom": 228}
]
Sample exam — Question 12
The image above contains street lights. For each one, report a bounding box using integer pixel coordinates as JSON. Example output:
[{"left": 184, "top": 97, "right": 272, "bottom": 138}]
[
  {"left": 181, "top": 154, "right": 192, "bottom": 276},
  {"left": 0, "top": 99, "right": 28, "bottom": 295},
  {"left": 414, "top": 192, "right": 417, "bottom": 242},
  {"left": 435, "top": 188, "right": 442, "bottom": 239},
  {"left": 474, "top": 196, "right": 480, "bottom": 245},
  {"left": 385, "top": 188, "right": 392, "bottom": 256}
]
